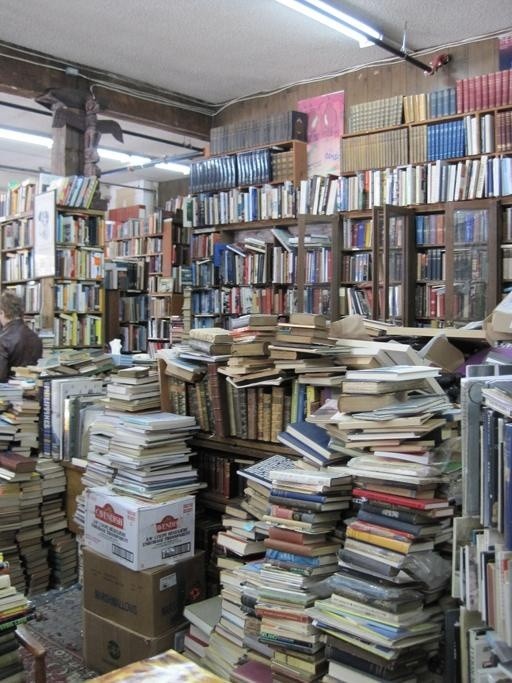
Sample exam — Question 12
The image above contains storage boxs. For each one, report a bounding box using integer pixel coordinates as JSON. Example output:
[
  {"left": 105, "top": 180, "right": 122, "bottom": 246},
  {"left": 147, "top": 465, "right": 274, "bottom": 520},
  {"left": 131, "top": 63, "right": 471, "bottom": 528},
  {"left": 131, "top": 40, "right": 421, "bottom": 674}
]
[{"left": 79, "top": 492, "right": 209, "bottom": 675}]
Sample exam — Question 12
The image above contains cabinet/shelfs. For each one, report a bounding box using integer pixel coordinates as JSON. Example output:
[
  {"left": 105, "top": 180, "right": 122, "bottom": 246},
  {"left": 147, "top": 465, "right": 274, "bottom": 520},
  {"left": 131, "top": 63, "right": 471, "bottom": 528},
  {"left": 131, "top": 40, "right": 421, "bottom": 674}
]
[{"left": 0, "top": 104, "right": 511, "bottom": 518}]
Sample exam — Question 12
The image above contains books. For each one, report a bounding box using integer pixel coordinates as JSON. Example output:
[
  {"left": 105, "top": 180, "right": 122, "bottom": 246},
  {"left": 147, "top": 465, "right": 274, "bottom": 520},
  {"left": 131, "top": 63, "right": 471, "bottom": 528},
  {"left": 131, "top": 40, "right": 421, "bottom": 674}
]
[
  {"left": 46, "top": 71, "right": 512, "bottom": 346},
  {"left": 0, "top": 182, "right": 44, "bottom": 333},
  {"left": 1, "top": 315, "right": 512, "bottom": 683}
]
